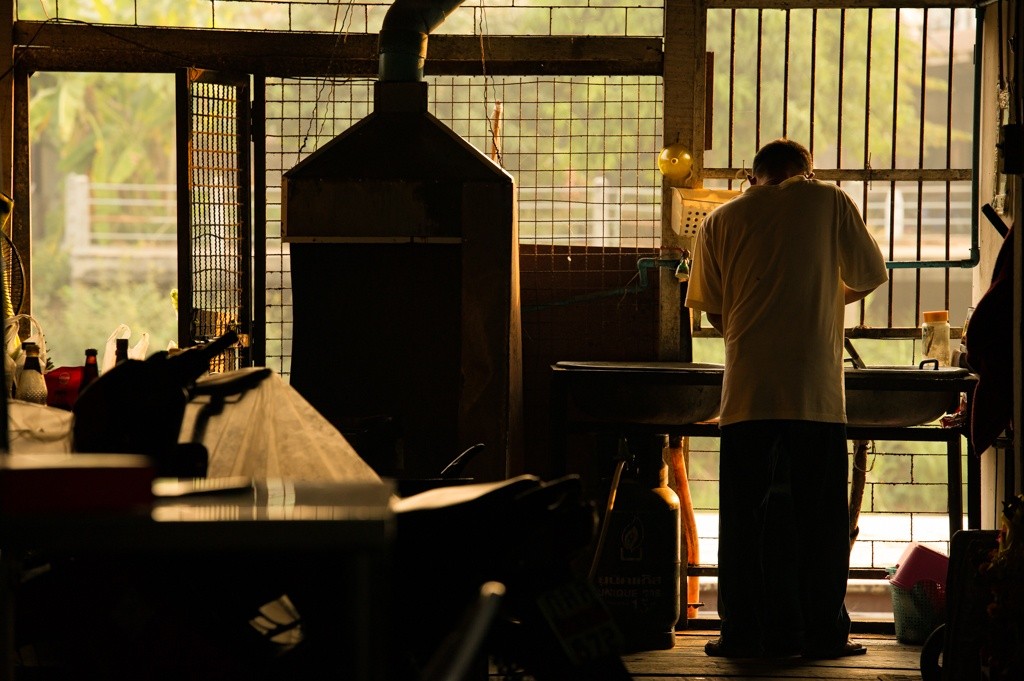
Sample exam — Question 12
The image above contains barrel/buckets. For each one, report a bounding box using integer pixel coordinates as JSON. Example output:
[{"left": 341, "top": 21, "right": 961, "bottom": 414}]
[{"left": 889, "top": 545, "right": 949, "bottom": 598}]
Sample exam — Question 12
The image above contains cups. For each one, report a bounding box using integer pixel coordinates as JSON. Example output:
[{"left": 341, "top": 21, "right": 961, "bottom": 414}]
[{"left": 961, "top": 306, "right": 975, "bottom": 347}]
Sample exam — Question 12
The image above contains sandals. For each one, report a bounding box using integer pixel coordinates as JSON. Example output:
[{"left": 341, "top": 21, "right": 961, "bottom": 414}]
[
  {"left": 844, "top": 642, "right": 866, "bottom": 654},
  {"left": 706, "top": 640, "right": 726, "bottom": 655}
]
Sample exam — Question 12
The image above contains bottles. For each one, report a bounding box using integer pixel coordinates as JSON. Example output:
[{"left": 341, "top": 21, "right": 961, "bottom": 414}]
[
  {"left": 921, "top": 310, "right": 952, "bottom": 368},
  {"left": 79, "top": 349, "right": 100, "bottom": 393},
  {"left": 14, "top": 342, "right": 48, "bottom": 406},
  {"left": 116, "top": 339, "right": 127, "bottom": 364}
]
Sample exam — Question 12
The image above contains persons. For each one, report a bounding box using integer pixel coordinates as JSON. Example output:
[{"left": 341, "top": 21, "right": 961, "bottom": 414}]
[{"left": 684, "top": 140, "right": 889, "bottom": 657}]
